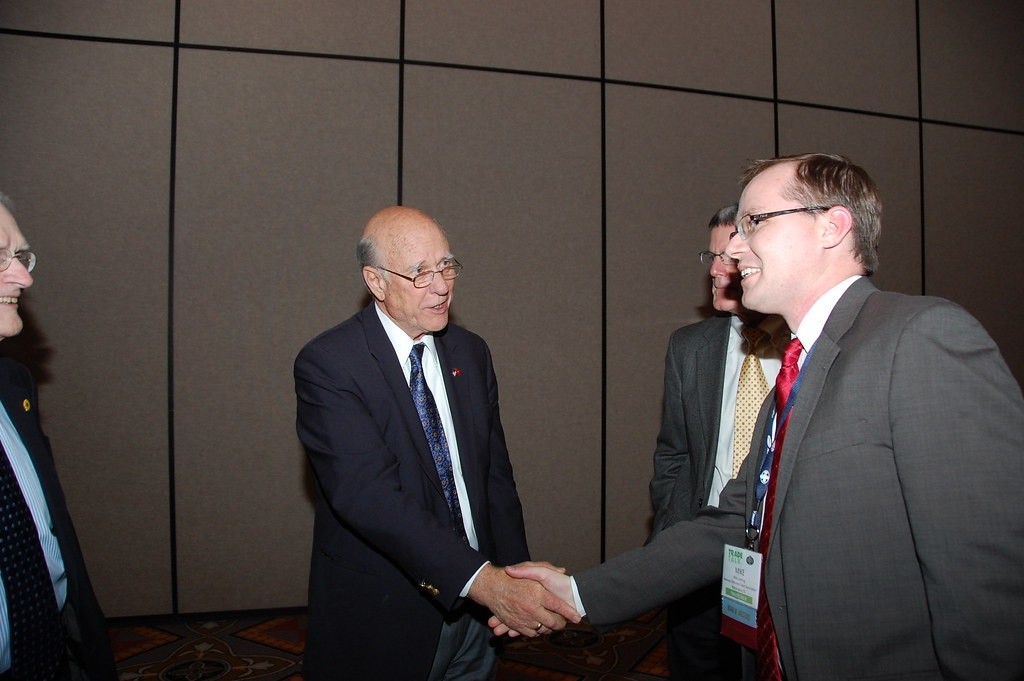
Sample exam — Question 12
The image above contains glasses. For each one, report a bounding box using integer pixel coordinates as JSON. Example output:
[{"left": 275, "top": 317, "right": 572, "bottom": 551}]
[
  {"left": 700, "top": 251, "right": 739, "bottom": 266},
  {"left": 730, "top": 201, "right": 844, "bottom": 242},
  {"left": 374, "top": 257, "right": 464, "bottom": 290},
  {"left": 0, "top": 248, "right": 36, "bottom": 273}
]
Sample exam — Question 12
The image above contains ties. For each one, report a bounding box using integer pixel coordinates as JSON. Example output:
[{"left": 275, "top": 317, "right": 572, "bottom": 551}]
[
  {"left": 732, "top": 327, "right": 769, "bottom": 481},
  {"left": 756, "top": 339, "right": 803, "bottom": 681},
  {"left": 0, "top": 442, "right": 66, "bottom": 681},
  {"left": 410, "top": 343, "right": 470, "bottom": 549}
]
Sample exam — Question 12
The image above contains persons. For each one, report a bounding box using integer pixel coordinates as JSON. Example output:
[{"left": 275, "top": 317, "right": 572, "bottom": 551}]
[
  {"left": 488, "top": 154, "right": 1024, "bottom": 681},
  {"left": 293, "top": 207, "right": 582, "bottom": 681},
  {"left": 650, "top": 203, "right": 791, "bottom": 681},
  {"left": 1, "top": 191, "right": 116, "bottom": 681}
]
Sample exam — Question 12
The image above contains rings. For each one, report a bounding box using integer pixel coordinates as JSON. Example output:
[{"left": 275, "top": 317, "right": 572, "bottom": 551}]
[{"left": 535, "top": 623, "right": 543, "bottom": 630}]
